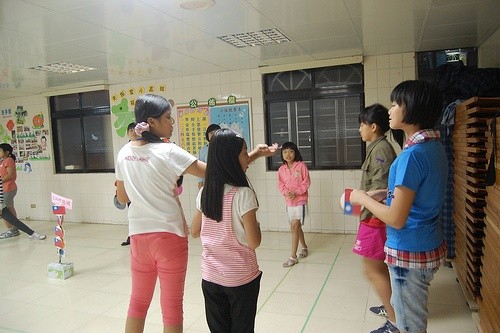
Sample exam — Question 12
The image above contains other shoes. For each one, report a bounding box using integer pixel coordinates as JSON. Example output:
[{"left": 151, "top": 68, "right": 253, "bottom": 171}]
[
  {"left": 29, "top": 232, "right": 46, "bottom": 240},
  {"left": 121, "top": 236, "right": 130, "bottom": 246},
  {"left": 0, "top": 226, "right": 20, "bottom": 239}
]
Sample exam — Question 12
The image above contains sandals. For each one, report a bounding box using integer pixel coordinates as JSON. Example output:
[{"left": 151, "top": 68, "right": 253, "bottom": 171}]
[
  {"left": 299, "top": 245, "right": 309, "bottom": 258},
  {"left": 282, "top": 255, "right": 299, "bottom": 268}
]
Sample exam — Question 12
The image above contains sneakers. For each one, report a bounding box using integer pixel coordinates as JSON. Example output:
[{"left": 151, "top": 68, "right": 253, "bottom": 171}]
[
  {"left": 367, "top": 304, "right": 388, "bottom": 317},
  {"left": 370, "top": 319, "right": 400, "bottom": 333}
]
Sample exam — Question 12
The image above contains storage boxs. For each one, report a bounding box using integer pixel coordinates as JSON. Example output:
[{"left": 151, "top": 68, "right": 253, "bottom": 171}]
[{"left": 47, "top": 260, "right": 76, "bottom": 280}]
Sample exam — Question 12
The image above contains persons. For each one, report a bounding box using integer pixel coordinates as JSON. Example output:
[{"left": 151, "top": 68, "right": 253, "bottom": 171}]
[
  {"left": 349, "top": 80, "right": 449, "bottom": 333},
  {"left": 115, "top": 93, "right": 285, "bottom": 333},
  {"left": 352, "top": 102, "right": 398, "bottom": 333},
  {"left": 0, "top": 143, "right": 48, "bottom": 240},
  {"left": 278, "top": 141, "right": 309, "bottom": 267}
]
